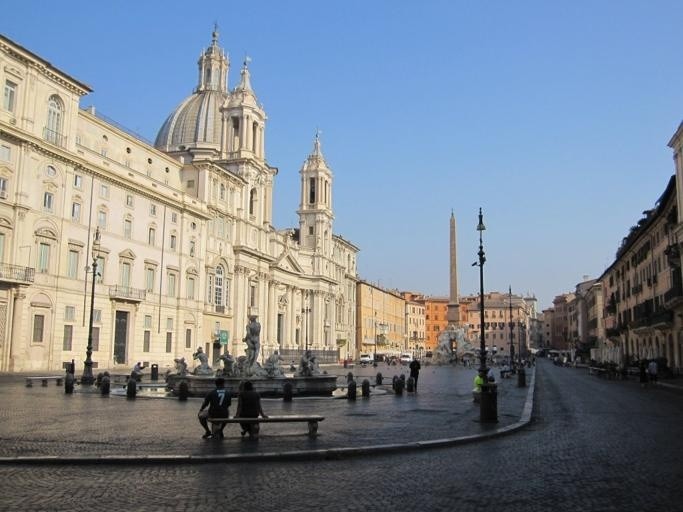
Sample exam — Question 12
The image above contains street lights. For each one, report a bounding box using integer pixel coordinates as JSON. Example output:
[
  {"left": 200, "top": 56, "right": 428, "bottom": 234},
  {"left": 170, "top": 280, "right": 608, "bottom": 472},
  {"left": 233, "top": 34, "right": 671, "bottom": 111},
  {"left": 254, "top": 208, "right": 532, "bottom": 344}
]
[
  {"left": 477, "top": 207, "right": 490, "bottom": 375},
  {"left": 509, "top": 286, "right": 514, "bottom": 364},
  {"left": 81, "top": 226, "right": 101, "bottom": 384}
]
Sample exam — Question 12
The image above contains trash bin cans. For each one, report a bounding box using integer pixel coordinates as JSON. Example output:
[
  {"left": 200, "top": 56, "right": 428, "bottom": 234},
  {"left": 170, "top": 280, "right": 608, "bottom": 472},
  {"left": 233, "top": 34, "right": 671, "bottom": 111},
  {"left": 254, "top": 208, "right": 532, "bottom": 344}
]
[
  {"left": 518, "top": 369, "right": 525, "bottom": 387},
  {"left": 479, "top": 384, "right": 497, "bottom": 418},
  {"left": 151, "top": 365, "right": 158, "bottom": 381}
]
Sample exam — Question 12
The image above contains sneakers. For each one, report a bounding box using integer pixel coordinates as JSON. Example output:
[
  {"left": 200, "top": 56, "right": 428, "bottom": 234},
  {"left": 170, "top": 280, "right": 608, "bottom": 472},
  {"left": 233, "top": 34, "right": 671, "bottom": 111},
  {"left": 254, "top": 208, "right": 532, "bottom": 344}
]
[
  {"left": 240, "top": 431, "right": 246, "bottom": 436},
  {"left": 201, "top": 431, "right": 212, "bottom": 438}
]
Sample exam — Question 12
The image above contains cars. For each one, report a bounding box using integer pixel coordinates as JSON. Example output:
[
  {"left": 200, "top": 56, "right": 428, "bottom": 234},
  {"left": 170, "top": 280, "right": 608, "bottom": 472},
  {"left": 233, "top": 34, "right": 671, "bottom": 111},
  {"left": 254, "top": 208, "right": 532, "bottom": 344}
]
[
  {"left": 401, "top": 353, "right": 412, "bottom": 362},
  {"left": 360, "top": 353, "right": 373, "bottom": 364}
]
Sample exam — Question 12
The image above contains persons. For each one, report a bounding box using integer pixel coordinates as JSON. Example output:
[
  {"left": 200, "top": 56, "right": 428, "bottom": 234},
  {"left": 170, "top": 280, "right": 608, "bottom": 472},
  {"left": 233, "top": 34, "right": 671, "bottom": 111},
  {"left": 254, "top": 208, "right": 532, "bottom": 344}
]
[
  {"left": 409, "top": 360, "right": 420, "bottom": 390},
  {"left": 192, "top": 346, "right": 209, "bottom": 370},
  {"left": 639, "top": 358, "right": 659, "bottom": 385},
  {"left": 500, "top": 353, "right": 626, "bottom": 379},
  {"left": 198, "top": 378, "right": 231, "bottom": 438},
  {"left": 133, "top": 362, "right": 141, "bottom": 372},
  {"left": 220, "top": 352, "right": 235, "bottom": 375},
  {"left": 290, "top": 360, "right": 296, "bottom": 371},
  {"left": 298, "top": 350, "right": 313, "bottom": 376},
  {"left": 471, "top": 369, "right": 485, "bottom": 404},
  {"left": 175, "top": 358, "right": 187, "bottom": 373},
  {"left": 268, "top": 349, "right": 285, "bottom": 375},
  {"left": 233, "top": 380, "right": 269, "bottom": 437},
  {"left": 242, "top": 315, "right": 261, "bottom": 366}
]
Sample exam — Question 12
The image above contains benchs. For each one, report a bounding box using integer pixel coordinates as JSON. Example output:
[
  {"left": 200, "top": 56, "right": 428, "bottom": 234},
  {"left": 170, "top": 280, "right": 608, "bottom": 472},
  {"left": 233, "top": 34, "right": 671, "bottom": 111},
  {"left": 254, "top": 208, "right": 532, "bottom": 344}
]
[{"left": 208, "top": 415, "right": 324, "bottom": 437}]
